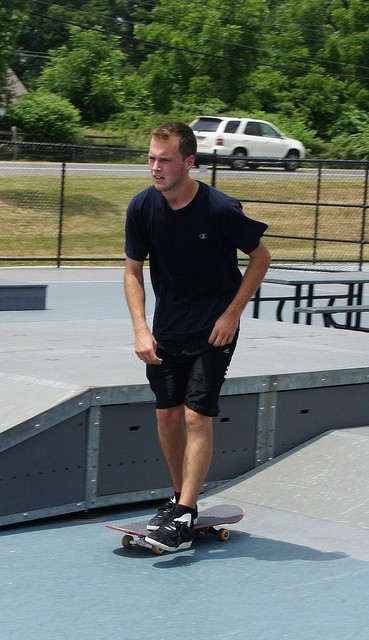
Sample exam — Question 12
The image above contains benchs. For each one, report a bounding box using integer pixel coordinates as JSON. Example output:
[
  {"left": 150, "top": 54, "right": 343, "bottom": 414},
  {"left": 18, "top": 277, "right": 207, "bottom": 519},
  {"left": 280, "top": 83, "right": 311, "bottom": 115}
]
[
  {"left": 296, "top": 304, "right": 369, "bottom": 330},
  {"left": 248, "top": 290, "right": 363, "bottom": 321}
]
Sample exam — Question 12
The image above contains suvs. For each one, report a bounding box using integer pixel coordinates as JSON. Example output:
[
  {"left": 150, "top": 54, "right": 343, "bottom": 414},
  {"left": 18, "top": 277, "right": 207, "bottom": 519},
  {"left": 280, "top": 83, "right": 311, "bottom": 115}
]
[{"left": 188, "top": 116, "right": 305, "bottom": 169}]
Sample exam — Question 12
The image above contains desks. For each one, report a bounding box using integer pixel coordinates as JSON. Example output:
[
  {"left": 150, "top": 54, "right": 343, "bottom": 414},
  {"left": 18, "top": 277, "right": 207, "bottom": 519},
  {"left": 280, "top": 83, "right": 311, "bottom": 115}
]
[{"left": 256, "top": 271, "right": 367, "bottom": 286}]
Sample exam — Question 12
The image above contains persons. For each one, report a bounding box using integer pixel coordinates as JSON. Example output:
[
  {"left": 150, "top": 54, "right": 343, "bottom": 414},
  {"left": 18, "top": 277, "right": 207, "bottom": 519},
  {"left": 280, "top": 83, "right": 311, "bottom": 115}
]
[{"left": 124, "top": 124, "right": 270, "bottom": 551}]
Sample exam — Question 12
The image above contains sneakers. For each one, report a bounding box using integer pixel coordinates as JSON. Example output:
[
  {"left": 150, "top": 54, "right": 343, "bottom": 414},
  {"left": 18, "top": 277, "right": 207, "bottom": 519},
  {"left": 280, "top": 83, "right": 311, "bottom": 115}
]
[
  {"left": 147, "top": 496, "right": 198, "bottom": 530},
  {"left": 144, "top": 507, "right": 192, "bottom": 552}
]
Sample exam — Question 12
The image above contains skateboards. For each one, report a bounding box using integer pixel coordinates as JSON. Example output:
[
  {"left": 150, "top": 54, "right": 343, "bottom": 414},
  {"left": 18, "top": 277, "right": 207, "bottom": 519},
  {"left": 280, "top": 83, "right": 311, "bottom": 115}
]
[{"left": 105, "top": 504, "right": 244, "bottom": 555}]
